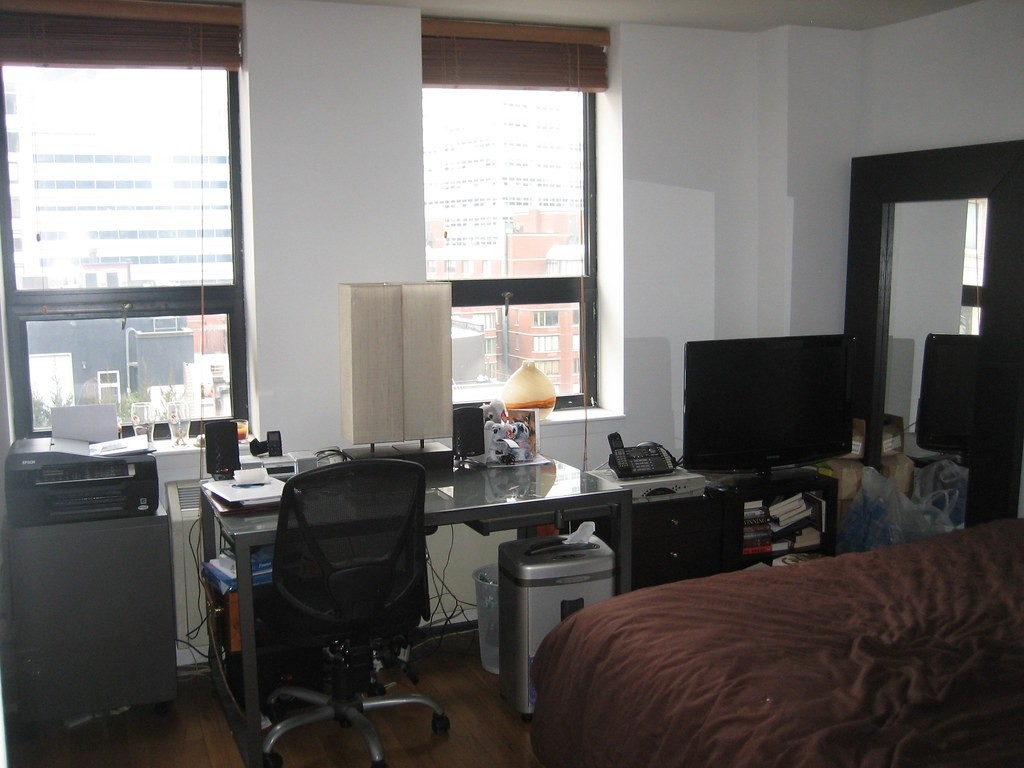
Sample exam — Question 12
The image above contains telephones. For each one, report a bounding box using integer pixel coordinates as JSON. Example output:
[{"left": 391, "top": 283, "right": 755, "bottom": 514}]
[{"left": 607, "top": 431, "right": 676, "bottom": 481}]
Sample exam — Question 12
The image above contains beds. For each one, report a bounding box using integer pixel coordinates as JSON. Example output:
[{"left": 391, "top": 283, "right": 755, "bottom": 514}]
[{"left": 519, "top": 519, "right": 1024, "bottom": 767}]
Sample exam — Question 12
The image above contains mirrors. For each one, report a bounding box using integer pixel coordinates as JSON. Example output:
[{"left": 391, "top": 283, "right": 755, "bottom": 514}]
[{"left": 883, "top": 197, "right": 988, "bottom": 466}]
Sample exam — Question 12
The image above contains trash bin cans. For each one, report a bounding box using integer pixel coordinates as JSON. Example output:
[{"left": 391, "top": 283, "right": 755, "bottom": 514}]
[{"left": 473, "top": 563, "right": 500, "bottom": 675}]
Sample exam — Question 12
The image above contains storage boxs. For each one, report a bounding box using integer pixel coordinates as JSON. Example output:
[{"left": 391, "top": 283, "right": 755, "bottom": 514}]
[
  {"left": 199, "top": 563, "right": 242, "bottom": 652},
  {"left": 818, "top": 416, "right": 915, "bottom": 543}
]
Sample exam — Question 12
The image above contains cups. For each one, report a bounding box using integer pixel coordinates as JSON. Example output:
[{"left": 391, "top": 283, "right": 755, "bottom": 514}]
[
  {"left": 168, "top": 402, "right": 191, "bottom": 449},
  {"left": 231, "top": 419, "right": 249, "bottom": 440},
  {"left": 131, "top": 402, "right": 155, "bottom": 448}
]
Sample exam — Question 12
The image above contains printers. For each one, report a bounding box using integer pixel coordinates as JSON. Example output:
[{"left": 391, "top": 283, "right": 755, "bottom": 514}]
[{"left": 3, "top": 402, "right": 159, "bottom": 526}]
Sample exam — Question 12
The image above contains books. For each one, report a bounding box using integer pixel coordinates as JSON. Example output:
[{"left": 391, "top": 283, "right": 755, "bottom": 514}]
[
  {"left": 201, "top": 547, "right": 274, "bottom": 590},
  {"left": 742, "top": 492, "right": 827, "bottom": 554},
  {"left": 201, "top": 476, "right": 304, "bottom": 517}
]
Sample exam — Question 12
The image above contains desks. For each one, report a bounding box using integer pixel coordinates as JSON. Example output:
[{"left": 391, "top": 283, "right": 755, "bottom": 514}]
[{"left": 200, "top": 454, "right": 634, "bottom": 768}]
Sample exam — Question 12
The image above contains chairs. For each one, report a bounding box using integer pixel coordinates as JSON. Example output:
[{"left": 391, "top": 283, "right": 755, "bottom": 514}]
[{"left": 251, "top": 459, "right": 453, "bottom": 768}]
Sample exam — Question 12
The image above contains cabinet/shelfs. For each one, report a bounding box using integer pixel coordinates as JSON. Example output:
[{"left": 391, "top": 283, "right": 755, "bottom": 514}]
[
  {"left": 629, "top": 486, "right": 735, "bottom": 595},
  {"left": 727, "top": 474, "right": 842, "bottom": 569}
]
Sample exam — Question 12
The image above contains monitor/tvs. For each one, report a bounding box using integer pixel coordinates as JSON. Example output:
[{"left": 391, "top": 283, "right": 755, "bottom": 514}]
[
  {"left": 916, "top": 334, "right": 981, "bottom": 457},
  {"left": 682, "top": 334, "right": 856, "bottom": 488}
]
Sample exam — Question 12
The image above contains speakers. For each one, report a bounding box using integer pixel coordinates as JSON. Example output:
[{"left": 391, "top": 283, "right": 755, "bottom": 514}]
[
  {"left": 203, "top": 421, "right": 240, "bottom": 482},
  {"left": 451, "top": 406, "right": 486, "bottom": 470}
]
